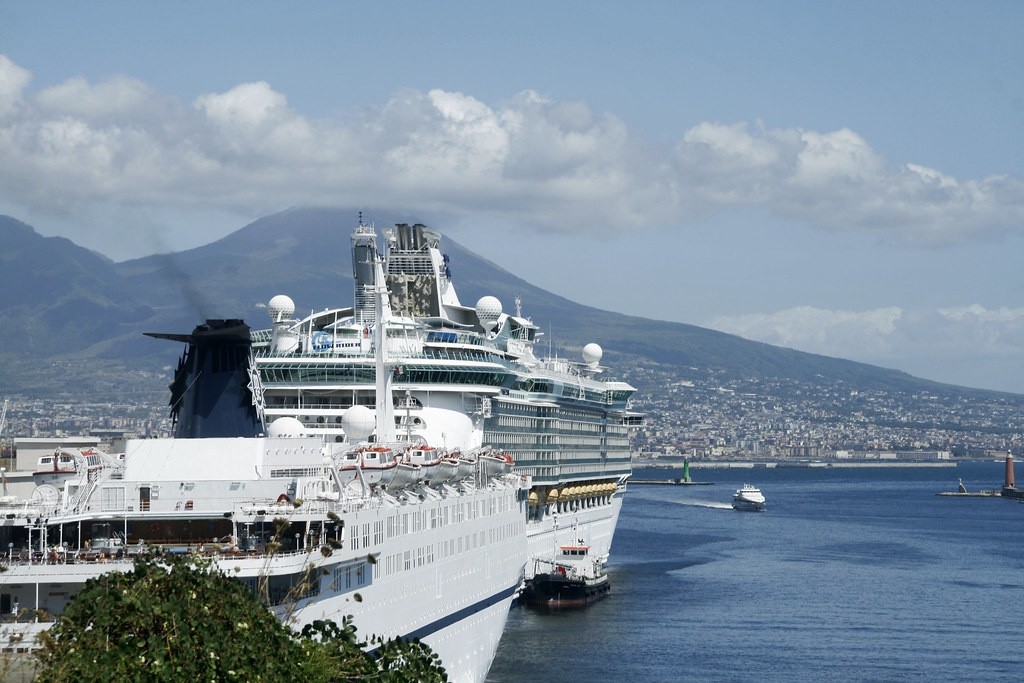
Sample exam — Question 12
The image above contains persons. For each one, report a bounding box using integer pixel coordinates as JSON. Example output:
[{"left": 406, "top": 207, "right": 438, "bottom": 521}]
[{"left": 18, "top": 539, "right": 255, "bottom": 561}]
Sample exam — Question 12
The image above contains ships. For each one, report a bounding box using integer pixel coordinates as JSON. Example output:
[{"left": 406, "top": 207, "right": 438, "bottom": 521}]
[
  {"left": 0, "top": 261, "right": 538, "bottom": 683},
  {"left": 246, "top": 206, "right": 648, "bottom": 606}
]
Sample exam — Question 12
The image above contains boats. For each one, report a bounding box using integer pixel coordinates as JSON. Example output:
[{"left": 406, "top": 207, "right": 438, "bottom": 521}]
[
  {"left": 334, "top": 440, "right": 515, "bottom": 487},
  {"left": 732, "top": 482, "right": 767, "bottom": 512},
  {"left": 938, "top": 482, "right": 1002, "bottom": 497},
  {"left": 523, "top": 510, "right": 610, "bottom": 610}
]
